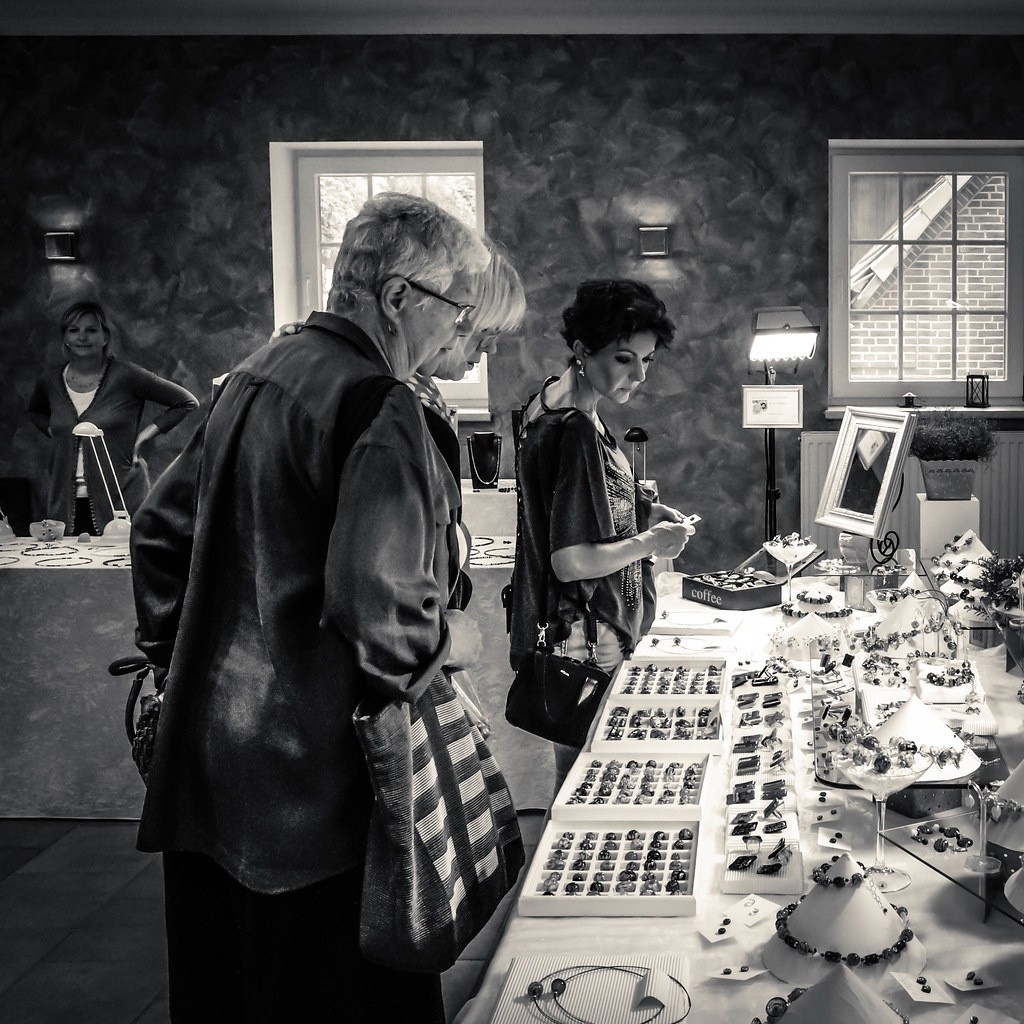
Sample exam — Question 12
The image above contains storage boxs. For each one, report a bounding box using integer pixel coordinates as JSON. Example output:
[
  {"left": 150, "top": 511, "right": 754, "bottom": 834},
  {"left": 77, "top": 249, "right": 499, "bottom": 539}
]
[
  {"left": 682, "top": 544, "right": 825, "bottom": 610},
  {"left": 517, "top": 633, "right": 732, "bottom": 917},
  {"left": 460, "top": 477, "right": 518, "bottom": 538}
]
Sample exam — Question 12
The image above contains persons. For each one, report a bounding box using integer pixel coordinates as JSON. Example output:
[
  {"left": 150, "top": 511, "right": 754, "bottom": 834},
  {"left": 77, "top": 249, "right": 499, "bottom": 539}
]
[
  {"left": 268, "top": 251, "right": 525, "bottom": 611},
  {"left": 107, "top": 192, "right": 491, "bottom": 1024},
  {"left": 501, "top": 279, "right": 696, "bottom": 839},
  {"left": 26, "top": 301, "right": 200, "bottom": 537}
]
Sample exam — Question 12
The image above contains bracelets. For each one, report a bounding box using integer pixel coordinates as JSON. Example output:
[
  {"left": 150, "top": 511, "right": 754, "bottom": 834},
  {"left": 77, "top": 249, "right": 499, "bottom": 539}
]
[
  {"left": 911, "top": 823, "right": 973, "bottom": 854},
  {"left": 861, "top": 653, "right": 899, "bottom": 674},
  {"left": 927, "top": 666, "right": 974, "bottom": 688},
  {"left": 864, "top": 666, "right": 907, "bottom": 688},
  {"left": 811, "top": 854, "right": 888, "bottom": 915}
]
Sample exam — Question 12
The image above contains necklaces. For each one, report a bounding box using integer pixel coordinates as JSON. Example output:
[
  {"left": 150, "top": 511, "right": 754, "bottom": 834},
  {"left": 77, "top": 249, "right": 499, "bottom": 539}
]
[
  {"left": 774, "top": 894, "right": 913, "bottom": 966},
  {"left": 69, "top": 365, "right": 103, "bottom": 389},
  {"left": 0, "top": 519, "right": 132, "bottom": 567},
  {"left": 462, "top": 435, "right": 517, "bottom": 566},
  {"left": 525, "top": 964, "right": 691, "bottom": 1024},
  {"left": 980, "top": 788, "right": 1024, "bottom": 822},
  {"left": 855, "top": 720, "right": 974, "bottom": 766},
  {"left": 862, "top": 614, "right": 963, "bottom": 663},
  {"left": 780, "top": 534, "right": 1024, "bottom": 618},
  {"left": 751, "top": 985, "right": 910, "bottom": 1024},
  {"left": 650, "top": 607, "right": 728, "bottom": 656}
]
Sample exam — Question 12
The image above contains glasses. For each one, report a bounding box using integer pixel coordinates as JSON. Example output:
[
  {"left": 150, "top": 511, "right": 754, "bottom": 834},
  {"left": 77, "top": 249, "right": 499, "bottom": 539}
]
[
  {"left": 471, "top": 321, "right": 503, "bottom": 349},
  {"left": 379, "top": 274, "right": 475, "bottom": 324}
]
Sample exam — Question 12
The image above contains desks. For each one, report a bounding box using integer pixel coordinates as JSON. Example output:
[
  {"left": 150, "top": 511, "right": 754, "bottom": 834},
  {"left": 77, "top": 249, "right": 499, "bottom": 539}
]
[
  {"left": 0, "top": 537, "right": 601, "bottom": 819},
  {"left": 456, "top": 572, "right": 1024, "bottom": 1024}
]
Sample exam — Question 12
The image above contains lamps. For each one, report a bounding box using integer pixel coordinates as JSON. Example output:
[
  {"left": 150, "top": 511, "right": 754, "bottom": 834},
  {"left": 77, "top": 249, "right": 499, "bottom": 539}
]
[
  {"left": 746, "top": 306, "right": 819, "bottom": 577},
  {"left": 72, "top": 423, "right": 133, "bottom": 547}
]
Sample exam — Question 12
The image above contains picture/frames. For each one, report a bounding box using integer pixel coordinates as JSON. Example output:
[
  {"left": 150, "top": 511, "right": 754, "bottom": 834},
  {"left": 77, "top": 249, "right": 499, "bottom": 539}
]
[
  {"left": 815, "top": 405, "right": 918, "bottom": 541},
  {"left": 742, "top": 385, "right": 803, "bottom": 429}
]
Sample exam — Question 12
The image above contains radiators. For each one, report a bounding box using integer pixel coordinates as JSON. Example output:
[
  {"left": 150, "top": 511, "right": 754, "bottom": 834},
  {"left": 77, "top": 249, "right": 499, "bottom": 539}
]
[{"left": 800, "top": 432, "right": 1024, "bottom": 576}]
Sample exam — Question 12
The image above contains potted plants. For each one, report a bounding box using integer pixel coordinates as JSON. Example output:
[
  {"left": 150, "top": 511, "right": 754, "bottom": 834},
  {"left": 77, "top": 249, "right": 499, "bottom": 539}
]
[{"left": 910, "top": 418, "right": 997, "bottom": 500}]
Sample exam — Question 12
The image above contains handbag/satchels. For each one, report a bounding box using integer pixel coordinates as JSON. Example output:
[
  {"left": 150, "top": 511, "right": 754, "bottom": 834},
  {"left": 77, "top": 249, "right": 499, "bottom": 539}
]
[
  {"left": 107, "top": 655, "right": 162, "bottom": 788},
  {"left": 506, "top": 646, "right": 611, "bottom": 749},
  {"left": 353, "top": 666, "right": 525, "bottom": 977}
]
